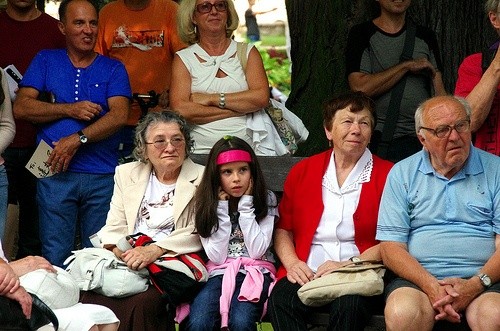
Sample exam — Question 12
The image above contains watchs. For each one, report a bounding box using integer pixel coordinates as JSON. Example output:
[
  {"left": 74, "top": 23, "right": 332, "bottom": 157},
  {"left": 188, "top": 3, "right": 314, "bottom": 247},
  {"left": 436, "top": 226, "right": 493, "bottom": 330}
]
[
  {"left": 350, "top": 255, "right": 361, "bottom": 263},
  {"left": 76, "top": 130, "right": 87, "bottom": 145},
  {"left": 476, "top": 272, "right": 492, "bottom": 289}
]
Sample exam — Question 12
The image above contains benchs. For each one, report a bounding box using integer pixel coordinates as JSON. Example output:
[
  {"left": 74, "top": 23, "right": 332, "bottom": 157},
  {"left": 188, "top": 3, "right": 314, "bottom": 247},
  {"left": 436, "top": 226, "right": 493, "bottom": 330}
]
[{"left": 189, "top": 152, "right": 387, "bottom": 326}]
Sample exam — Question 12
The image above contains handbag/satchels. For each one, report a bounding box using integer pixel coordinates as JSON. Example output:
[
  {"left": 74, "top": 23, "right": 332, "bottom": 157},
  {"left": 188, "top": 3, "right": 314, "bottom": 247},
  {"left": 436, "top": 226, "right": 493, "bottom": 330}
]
[
  {"left": 296, "top": 260, "right": 386, "bottom": 307},
  {"left": 19, "top": 265, "right": 80, "bottom": 308},
  {"left": 235, "top": 41, "right": 298, "bottom": 156},
  {"left": 63, "top": 247, "right": 150, "bottom": 296}
]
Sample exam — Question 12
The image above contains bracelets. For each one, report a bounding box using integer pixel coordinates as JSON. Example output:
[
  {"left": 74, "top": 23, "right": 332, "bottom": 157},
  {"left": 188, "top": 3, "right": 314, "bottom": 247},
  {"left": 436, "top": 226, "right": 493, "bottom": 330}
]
[{"left": 219, "top": 92, "right": 225, "bottom": 107}]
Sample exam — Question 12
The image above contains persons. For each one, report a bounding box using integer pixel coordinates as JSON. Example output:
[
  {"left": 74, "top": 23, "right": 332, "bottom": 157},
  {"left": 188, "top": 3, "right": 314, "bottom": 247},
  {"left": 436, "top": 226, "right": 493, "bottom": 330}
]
[
  {"left": 245, "top": 0, "right": 277, "bottom": 43},
  {"left": 12, "top": 0, "right": 132, "bottom": 269},
  {"left": 0, "top": 0, "right": 67, "bottom": 263},
  {"left": 375, "top": 95, "right": 500, "bottom": 331},
  {"left": 178, "top": 136, "right": 280, "bottom": 331},
  {"left": 453, "top": 0, "right": 500, "bottom": 157},
  {"left": 169, "top": 0, "right": 269, "bottom": 154},
  {"left": 346, "top": 0, "right": 447, "bottom": 165},
  {"left": 81, "top": 111, "right": 206, "bottom": 331},
  {"left": 0, "top": 242, "right": 120, "bottom": 331},
  {"left": 268, "top": 91, "right": 393, "bottom": 331},
  {"left": 0, "top": 67, "right": 15, "bottom": 240},
  {"left": 96, "top": 0, "right": 192, "bottom": 171}
]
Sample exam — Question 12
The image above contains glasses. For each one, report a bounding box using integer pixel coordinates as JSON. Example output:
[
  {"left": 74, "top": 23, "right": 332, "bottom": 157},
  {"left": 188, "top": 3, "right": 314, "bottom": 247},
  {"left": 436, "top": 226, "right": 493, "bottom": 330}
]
[
  {"left": 194, "top": 1, "right": 229, "bottom": 13},
  {"left": 419, "top": 116, "right": 472, "bottom": 138},
  {"left": 144, "top": 136, "right": 187, "bottom": 150}
]
